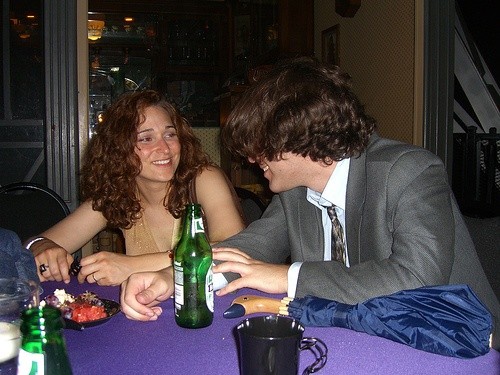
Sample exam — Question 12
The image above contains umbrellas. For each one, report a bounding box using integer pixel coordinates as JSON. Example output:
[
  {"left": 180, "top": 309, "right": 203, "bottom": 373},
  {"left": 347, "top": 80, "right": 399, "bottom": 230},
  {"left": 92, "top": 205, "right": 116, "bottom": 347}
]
[{"left": 223, "top": 283, "right": 495, "bottom": 359}]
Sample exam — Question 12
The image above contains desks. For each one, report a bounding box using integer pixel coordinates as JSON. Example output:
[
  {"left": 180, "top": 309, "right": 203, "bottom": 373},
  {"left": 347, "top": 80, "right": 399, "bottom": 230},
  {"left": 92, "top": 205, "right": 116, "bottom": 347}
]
[{"left": 0, "top": 274, "right": 500, "bottom": 375}]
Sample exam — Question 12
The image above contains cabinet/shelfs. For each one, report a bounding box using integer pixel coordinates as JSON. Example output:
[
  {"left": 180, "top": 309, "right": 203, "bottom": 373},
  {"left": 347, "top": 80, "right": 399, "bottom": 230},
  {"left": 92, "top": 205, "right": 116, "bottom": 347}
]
[{"left": 88, "top": 0, "right": 230, "bottom": 128}]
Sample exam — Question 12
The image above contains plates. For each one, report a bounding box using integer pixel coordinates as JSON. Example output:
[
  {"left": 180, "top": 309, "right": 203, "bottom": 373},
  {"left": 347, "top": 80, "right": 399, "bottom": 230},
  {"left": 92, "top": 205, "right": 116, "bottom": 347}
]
[{"left": 62, "top": 298, "right": 123, "bottom": 331}]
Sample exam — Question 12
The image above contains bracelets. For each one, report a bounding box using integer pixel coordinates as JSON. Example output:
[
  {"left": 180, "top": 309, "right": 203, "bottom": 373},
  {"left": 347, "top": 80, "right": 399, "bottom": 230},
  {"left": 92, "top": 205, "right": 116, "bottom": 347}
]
[{"left": 26, "top": 236, "right": 46, "bottom": 250}]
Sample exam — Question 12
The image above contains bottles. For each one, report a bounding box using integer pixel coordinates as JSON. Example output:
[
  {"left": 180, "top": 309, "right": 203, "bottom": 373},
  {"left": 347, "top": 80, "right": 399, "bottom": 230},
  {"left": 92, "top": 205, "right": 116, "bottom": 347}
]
[
  {"left": 18, "top": 307, "right": 73, "bottom": 375},
  {"left": 173, "top": 203, "right": 214, "bottom": 328},
  {"left": 167, "top": 31, "right": 209, "bottom": 63}
]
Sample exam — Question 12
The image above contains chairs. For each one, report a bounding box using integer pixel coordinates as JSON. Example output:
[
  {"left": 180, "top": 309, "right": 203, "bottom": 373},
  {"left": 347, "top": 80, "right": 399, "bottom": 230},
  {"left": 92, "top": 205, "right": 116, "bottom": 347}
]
[{"left": 0, "top": 182, "right": 82, "bottom": 262}]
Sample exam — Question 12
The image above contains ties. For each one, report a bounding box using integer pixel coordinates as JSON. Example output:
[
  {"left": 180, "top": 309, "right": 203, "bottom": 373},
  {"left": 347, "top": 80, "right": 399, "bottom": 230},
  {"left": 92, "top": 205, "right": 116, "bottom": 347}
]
[{"left": 318, "top": 202, "right": 347, "bottom": 265}]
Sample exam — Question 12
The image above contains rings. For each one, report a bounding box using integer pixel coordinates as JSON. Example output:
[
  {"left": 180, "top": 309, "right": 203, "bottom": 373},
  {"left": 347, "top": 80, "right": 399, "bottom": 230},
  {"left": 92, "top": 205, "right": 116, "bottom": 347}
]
[
  {"left": 91, "top": 272, "right": 98, "bottom": 283},
  {"left": 39, "top": 264, "right": 49, "bottom": 275}
]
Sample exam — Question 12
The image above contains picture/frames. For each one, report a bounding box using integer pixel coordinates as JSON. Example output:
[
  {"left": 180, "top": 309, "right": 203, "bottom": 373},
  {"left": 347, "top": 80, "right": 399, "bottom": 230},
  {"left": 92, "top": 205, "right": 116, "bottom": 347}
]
[{"left": 321, "top": 22, "right": 340, "bottom": 64}]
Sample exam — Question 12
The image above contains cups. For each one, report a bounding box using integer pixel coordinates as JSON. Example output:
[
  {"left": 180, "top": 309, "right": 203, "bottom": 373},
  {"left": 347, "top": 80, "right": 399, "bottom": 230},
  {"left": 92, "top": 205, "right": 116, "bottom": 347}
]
[
  {"left": 0, "top": 321, "right": 21, "bottom": 375},
  {"left": 0, "top": 279, "right": 39, "bottom": 326},
  {"left": 237, "top": 316, "right": 328, "bottom": 375}
]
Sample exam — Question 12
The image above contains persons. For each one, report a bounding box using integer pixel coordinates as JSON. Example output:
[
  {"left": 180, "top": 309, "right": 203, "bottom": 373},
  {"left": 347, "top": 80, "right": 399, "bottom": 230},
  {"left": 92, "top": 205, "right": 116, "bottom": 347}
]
[
  {"left": 120, "top": 56, "right": 500, "bottom": 352},
  {"left": 23, "top": 90, "right": 246, "bottom": 288}
]
